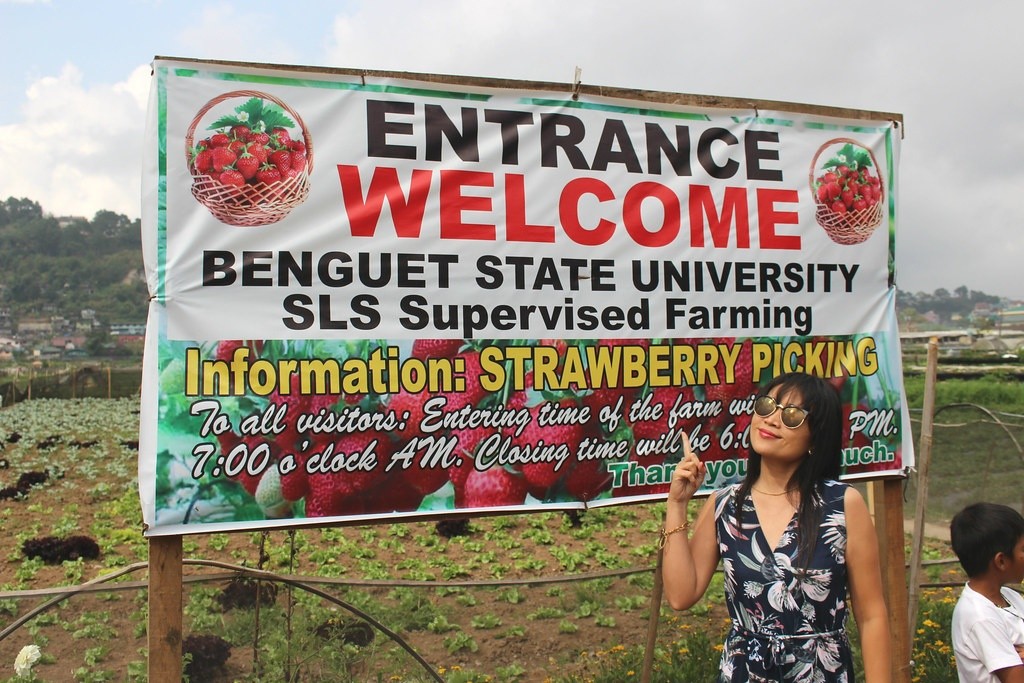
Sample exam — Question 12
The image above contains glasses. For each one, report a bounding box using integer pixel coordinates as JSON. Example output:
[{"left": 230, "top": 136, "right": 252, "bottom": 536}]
[{"left": 754, "top": 395, "right": 810, "bottom": 429}]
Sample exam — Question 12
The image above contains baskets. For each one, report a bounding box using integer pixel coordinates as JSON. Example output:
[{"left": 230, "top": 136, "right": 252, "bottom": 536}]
[
  {"left": 809, "top": 138, "right": 885, "bottom": 245},
  {"left": 186, "top": 91, "right": 314, "bottom": 227}
]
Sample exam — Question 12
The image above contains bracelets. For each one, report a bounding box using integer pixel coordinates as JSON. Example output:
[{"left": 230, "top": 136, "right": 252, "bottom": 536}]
[{"left": 663, "top": 520, "right": 690, "bottom": 537}]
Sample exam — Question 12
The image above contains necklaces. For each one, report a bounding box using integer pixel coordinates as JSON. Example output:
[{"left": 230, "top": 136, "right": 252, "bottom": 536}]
[
  {"left": 751, "top": 483, "right": 805, "bottom": 497},
  {"left": 987, "top": 593, "right": 1024, "bottom": 621}
]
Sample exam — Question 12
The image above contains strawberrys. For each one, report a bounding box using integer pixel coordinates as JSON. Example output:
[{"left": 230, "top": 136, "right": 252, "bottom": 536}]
[
  {"left": 194, "top": 126, "right": 308, "bottom": 205},
  {"left": 814, "top": 165, "right": 882, "bottom": 228},
  {"left": 159, "top": 341, "right": 902, "bottom": 518}
]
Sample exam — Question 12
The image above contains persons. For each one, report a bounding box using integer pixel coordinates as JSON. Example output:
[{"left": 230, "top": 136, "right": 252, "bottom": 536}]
[
  {"left": 950, "top": 501, "right": 1024, "bottom": 683},
  {"left": 661, "top": 372, "right": 894, "bottom": 683}
]
